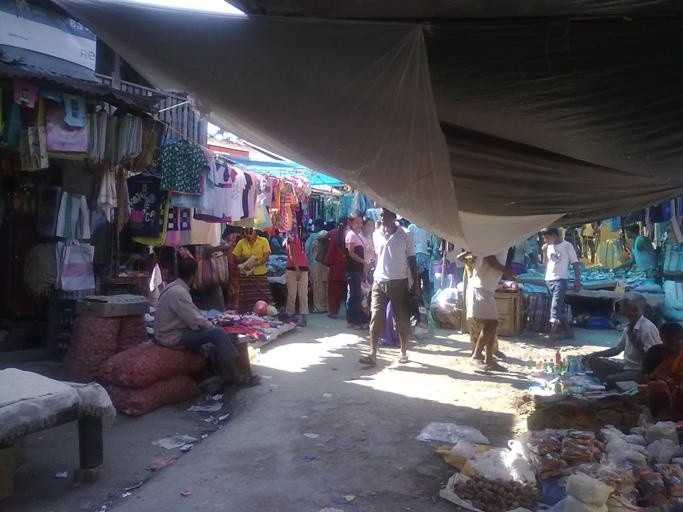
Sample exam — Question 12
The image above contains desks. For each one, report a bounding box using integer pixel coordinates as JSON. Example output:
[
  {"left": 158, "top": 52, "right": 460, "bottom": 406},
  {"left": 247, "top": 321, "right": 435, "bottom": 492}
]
[
  {"left": 514, "top": 276, "right": 664, "bottom": 327},
  {"left": 0, "top": 367, "right": 116, "bottom": 470}
]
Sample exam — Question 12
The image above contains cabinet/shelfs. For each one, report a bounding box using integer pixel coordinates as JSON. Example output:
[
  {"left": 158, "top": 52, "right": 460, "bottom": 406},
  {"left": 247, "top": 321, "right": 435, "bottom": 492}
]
[{"left": 0, "top": 213, "right": 45, "bottom": 320}]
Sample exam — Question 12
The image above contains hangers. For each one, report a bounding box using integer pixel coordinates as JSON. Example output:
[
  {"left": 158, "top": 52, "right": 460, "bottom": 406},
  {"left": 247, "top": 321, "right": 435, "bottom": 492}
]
[{"left": 176, "top": 132, "right": 192, "bottom": 146}]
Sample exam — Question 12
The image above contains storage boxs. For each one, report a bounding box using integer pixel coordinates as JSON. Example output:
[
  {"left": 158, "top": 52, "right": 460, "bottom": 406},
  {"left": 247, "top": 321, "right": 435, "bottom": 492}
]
[
  {"left": 76, "top": 293, "right": 147, "bottom": 317},
  {"left": 494, "top": 292, "right": 525, "bottom": 336},
  {"left": 0, "top": 445, "right": 14, "bottom": 503}
]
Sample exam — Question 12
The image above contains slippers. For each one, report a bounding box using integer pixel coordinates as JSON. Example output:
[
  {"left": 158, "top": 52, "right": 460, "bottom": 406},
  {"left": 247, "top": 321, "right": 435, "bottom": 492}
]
[
  {"left": 358, "top": 356, "right": 377, "bottom": 368},
  {"left": 396, "top": 356, "right": 409, "bottom": 364}
]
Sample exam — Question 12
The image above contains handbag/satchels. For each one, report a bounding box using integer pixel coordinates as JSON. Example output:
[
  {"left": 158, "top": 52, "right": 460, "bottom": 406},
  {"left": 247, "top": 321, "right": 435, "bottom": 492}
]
[
  {"left": 343, "top": 244, "right": 364, "bottom": 274},
  {"left": 55, "top": 239, "right": 97, "bottom": 292}
]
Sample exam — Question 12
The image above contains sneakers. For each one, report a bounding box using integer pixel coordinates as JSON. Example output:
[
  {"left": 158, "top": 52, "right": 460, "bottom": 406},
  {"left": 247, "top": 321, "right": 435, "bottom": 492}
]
[
  {"left": 232, "top": 373, "right": 262, "bottom": 389},
  {"left": 468, "top": 357, "right": 507, "bottom": 373}
]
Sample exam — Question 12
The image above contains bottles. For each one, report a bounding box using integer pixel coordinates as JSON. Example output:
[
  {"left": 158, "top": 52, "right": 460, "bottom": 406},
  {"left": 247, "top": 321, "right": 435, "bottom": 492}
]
[{"left": 527, "top": 349, "right": 568, "bottom": 375}]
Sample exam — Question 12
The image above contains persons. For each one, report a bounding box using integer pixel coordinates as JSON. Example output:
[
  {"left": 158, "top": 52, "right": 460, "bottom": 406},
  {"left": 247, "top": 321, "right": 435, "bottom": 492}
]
[{"left": 153, "top": 256, "right": 259, "bottom": 387}]
